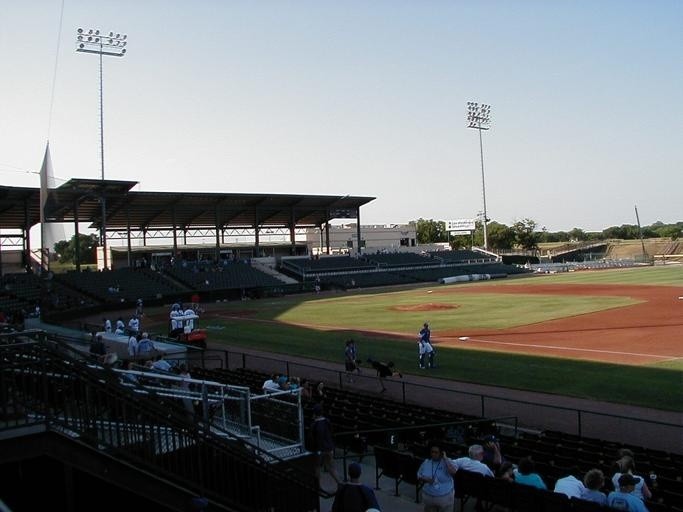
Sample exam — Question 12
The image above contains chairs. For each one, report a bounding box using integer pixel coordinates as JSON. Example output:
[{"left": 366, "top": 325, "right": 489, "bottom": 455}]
[
  {"left": 1, "top": 249, "right": 498, "bottom": 324},
  {"left": 169, "top": 364, "right": 681, "bottom": 511}
]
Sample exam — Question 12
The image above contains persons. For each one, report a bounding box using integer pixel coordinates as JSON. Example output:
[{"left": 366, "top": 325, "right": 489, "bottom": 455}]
[{"left": 0, "top": 252, "right": 683, "bottom": 512}]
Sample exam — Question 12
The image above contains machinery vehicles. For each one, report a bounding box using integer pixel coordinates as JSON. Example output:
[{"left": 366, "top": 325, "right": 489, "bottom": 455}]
[{"left": 166, "top": 309, "right": 208, "bottom": 348}]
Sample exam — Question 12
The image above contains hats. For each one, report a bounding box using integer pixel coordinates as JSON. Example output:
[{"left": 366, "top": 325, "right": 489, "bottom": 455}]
[
  {"left": 483, "top": 434, "right": 500, "bottom": 442},
  {"left": 618, "top": 475, "right": 640, "bottom": 486}
]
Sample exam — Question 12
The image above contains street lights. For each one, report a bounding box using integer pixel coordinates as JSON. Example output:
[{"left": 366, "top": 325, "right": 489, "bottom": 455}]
[
  {"left": 76, "top": 25, "right": 128, "bottom": 271},
  {"left": 463, "top": 99, "right": 493, "bottom": 251}
]
